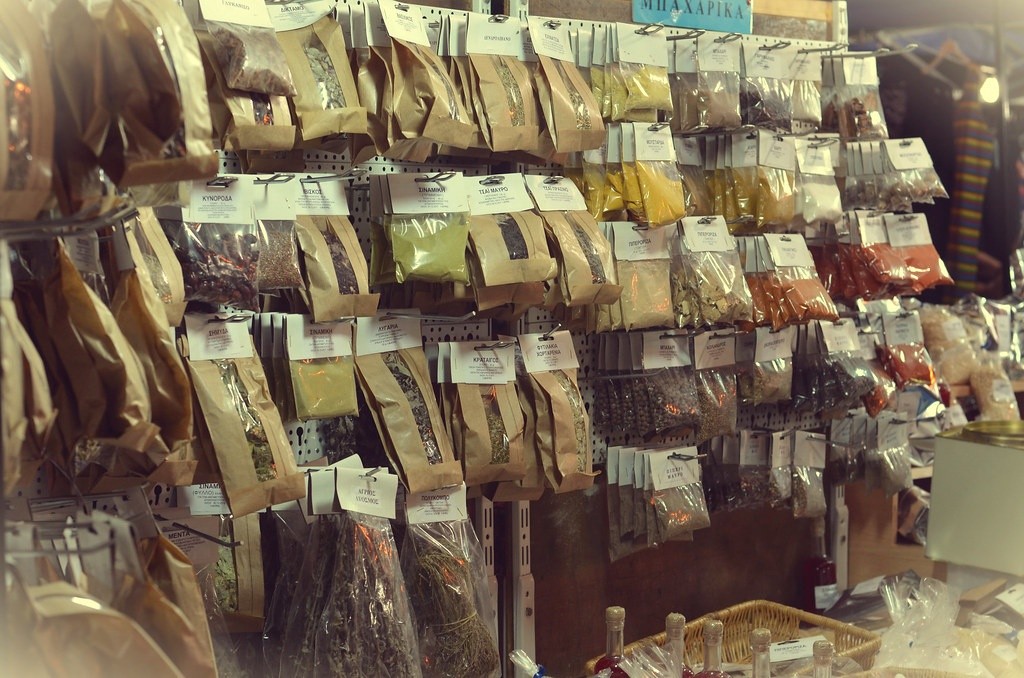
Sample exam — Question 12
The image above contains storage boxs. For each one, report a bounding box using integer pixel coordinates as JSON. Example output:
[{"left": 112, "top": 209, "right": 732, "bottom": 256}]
[
  {"left": 924, "top": 418, "right": 1024, "bottom": 578},
  {"left": 587, "top": 597, "right": 883, "bottom": 678}
]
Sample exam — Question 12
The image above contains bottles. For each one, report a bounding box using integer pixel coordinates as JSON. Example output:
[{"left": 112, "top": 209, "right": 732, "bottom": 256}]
[
  {"left": 663, "top": 614, "right": 696, "bottom": 677},
  {"left": 802, "top": 515, "right": 837, "bottom": 616},
  {"left": 694, "top": 618, "right": 731, "bottom": 678},
  {"left": 595, "top": 605, "right": 632, "bottom": 678},
  {"left": 812, "top": 640, "right": 835, "bottom": 678},
  {"left": 748, "top": 627, "right": 776, "bottom": 678}
]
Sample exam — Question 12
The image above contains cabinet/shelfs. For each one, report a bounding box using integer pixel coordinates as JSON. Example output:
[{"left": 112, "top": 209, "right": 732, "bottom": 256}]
[{"left": 0, "top": 0, "right": 848, "bottom": 678}]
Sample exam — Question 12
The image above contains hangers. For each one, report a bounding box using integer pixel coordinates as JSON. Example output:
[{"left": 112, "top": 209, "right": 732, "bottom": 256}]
[{"left": 919, "top": 24, "right": 986, "bottom": 91}]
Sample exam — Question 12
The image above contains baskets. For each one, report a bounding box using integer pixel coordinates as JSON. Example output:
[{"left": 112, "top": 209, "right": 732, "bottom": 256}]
[{"left": 585, "top": 595, "right": 882, "bottom": 676}]
